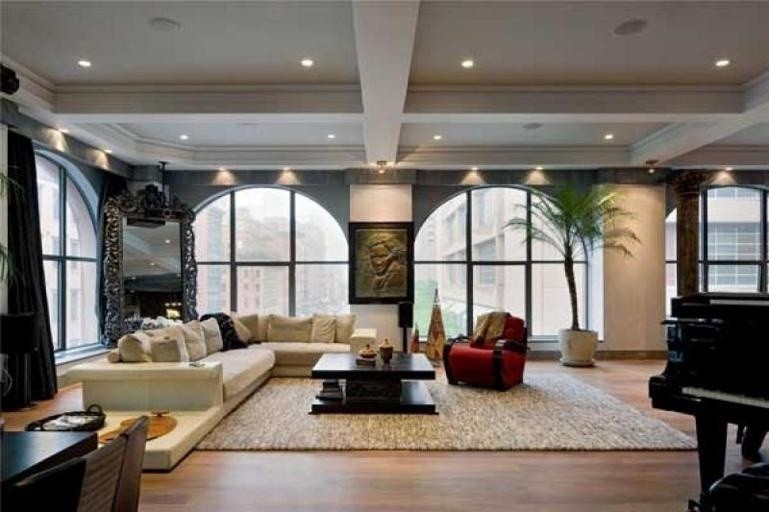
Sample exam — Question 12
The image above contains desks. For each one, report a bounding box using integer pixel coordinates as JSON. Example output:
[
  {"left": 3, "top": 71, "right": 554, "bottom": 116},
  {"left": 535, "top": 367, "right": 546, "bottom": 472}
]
[{"left": 0, "top": 424, "right": 100, "bottom": 510}]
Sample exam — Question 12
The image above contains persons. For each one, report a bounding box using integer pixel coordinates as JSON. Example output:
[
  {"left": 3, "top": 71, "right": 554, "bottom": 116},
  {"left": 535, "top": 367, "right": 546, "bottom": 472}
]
[{"left": 369, "top": 240, "right": 404, "bottom": 291}]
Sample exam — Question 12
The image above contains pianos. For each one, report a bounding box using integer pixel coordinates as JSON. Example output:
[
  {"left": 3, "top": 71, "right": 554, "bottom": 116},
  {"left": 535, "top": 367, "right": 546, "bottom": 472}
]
[{"left": 648, "top": 293, "right": 769, "bottom": 512}]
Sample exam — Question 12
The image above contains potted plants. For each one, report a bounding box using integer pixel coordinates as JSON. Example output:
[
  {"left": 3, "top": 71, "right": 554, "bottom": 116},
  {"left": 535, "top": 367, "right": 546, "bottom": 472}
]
[{"left": 506, "top": 178, "right": 642, "bottom": 367}]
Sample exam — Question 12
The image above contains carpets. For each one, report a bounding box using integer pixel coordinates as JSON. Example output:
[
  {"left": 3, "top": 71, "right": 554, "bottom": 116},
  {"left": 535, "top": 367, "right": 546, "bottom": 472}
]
[{"left": 193, "top": 371, "right": 698, "bottom": 451}]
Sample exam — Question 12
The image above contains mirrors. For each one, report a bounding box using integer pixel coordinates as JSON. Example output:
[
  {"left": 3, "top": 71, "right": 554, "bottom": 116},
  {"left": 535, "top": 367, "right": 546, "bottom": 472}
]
[{"left": 99, "top": 183, "right": 200, "bottom": 347}]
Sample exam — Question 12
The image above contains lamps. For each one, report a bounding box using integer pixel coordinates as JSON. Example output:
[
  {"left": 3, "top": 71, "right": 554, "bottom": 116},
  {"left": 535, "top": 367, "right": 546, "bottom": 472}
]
[
  {"left": 377, "top": 159, "right": 388, "bottom": 176},
  {"left": 611, "top": 21, "right": 650, "bottom": 38},
  {"left": 151, "top": 16, "right": 182, "bottom": 33},
  {"left": 645, "top": 158, "right": 660, "bottom": 176}
]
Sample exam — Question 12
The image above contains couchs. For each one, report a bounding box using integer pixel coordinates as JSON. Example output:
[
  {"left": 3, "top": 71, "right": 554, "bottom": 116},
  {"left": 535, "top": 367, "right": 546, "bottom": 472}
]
[
  {"left": 441, "top": 312, "right": 530, "bottom": 391},
  {"left": 62, "top": 305, "right": 377, "bottom": 470}
]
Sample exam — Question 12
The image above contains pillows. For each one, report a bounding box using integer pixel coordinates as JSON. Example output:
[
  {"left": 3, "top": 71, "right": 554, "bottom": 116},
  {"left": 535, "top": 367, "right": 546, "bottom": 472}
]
[{"left": 119, "top": 311, "right": 358, "bottom": 364}]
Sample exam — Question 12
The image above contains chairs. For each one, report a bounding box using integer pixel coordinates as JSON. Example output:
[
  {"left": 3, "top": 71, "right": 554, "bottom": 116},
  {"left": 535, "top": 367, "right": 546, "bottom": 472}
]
[{"left": 11, "top": 409, "right": 150, "bottom": 512}]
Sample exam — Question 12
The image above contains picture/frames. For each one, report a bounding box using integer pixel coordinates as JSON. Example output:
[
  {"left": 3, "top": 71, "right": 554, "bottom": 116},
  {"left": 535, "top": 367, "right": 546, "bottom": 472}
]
[{"left": 347, "top": 220, "right": 416, "bottom": 306}]
